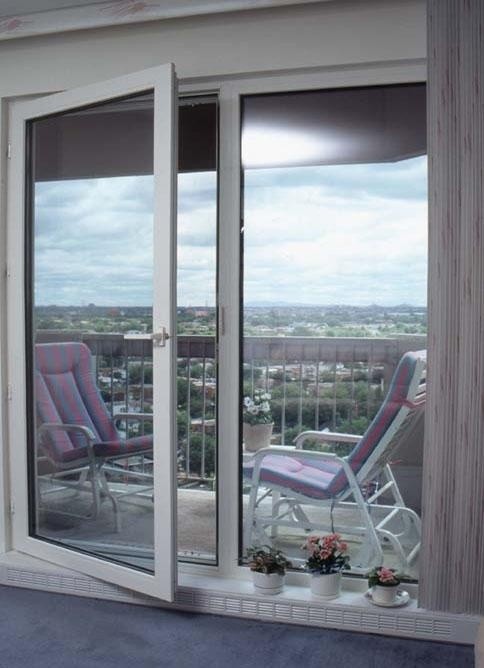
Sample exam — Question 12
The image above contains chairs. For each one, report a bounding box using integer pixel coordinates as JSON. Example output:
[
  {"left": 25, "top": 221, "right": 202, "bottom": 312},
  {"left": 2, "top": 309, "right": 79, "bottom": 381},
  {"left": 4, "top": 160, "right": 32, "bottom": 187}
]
[
  {"left": 31, "top": 342, "right": 153, "bottom": 534},
  {"left": 240, "top": 348, "right": 426, "bottom": 574}
]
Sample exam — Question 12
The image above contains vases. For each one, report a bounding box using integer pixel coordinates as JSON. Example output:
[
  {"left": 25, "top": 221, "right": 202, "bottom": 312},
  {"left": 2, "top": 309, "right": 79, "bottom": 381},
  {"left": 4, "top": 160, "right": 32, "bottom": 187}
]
[
  {"left": 242, "top": 423, "right": 273, "bottom": 454},
  {"left": 251, "top": 570, "right": 398, "bottom": 605}
]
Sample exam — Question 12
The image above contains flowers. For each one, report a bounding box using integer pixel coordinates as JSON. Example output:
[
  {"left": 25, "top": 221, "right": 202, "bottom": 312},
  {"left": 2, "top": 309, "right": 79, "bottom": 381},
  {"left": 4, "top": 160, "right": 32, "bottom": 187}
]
[
  {"left": 241, "top": 387, "right": 273, "bottom": 423},
  {"left": 245, "top": 532, "right": 403, "bottom": 587}
]
